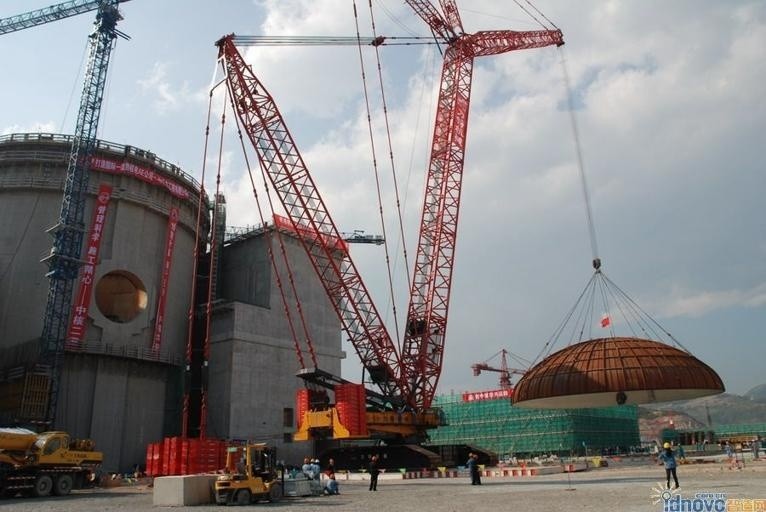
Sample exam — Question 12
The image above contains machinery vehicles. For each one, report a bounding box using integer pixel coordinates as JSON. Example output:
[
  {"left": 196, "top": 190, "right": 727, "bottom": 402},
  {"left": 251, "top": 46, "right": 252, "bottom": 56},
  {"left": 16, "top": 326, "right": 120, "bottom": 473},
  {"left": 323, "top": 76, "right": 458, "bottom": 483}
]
[{"left": 213, "top": 442, "right": 284, "bottom": 506}]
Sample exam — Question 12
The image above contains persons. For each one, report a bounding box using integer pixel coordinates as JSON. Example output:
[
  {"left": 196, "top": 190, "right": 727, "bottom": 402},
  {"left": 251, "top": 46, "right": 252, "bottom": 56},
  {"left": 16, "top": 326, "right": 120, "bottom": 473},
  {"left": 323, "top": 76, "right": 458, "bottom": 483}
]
[
  {"left": 725, "top": 440, "right": 735, "bottom": 458},
  {"left": 467, "top": 453, "right": 476, "bottom": 485},
  {"left": 599, "top": 444, "right": 636, "bottom": 455},
  {"left": 659, "top": 443, "right": 679, "bottom": 489},
  {"left": 678, "top": 444, "right": 686, "bottom": 461},
  {"left": 276, "top": 457, "right": 339, "bottom": 494},
  {"left": 473, "top": 454, "right": 482, "bottom": 485},
  {"left": 369, "top": 456, "right": 379, "bottom": 490},
  {"left": 751, "top": 439, "right": 760, "bottom": 458},
  {"left": 649, "top": 440, "right": 658, "bottom": 462}
]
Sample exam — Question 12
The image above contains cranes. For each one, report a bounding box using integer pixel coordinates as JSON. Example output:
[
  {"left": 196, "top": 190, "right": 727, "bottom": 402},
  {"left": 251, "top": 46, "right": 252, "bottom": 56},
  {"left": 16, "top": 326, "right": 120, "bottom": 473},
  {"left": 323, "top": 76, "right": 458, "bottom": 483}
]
[
  {"left": 211, "top": 0, "right": 568, "bottom": 448},
  {"left": 0, "top": 1, "right": 132, "bottom": 500}
]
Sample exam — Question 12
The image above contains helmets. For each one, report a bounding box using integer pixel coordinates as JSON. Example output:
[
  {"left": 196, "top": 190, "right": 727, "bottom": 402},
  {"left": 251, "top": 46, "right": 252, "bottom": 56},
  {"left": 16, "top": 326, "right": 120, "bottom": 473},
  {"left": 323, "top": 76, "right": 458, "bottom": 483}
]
[
  {"left": 469, "top": 453, "right": 478, "bottom": 459},
  {"left": 372, "top": 456, "right": 377, "bottom": 461},
  {"left": 664, "top": 443, "right": 670, "bottom": 449},
  {"left": 305, "top": 458, "right": 319, "bottom": 464},
  {"left": 330, "top": 474, "right": 335, "bottom": 478}
]
